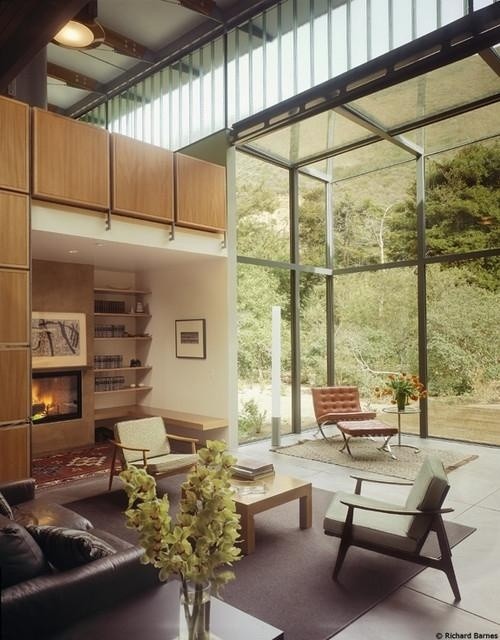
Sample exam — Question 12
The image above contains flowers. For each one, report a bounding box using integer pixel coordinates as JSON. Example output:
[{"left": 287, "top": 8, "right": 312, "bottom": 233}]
[{"left": 375, "top": 374, "right": 428, "bottom": 405}]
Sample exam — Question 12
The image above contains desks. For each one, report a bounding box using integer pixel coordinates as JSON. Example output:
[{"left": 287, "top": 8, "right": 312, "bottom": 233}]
[
  {"left": 95, "top": 406, "right": 227, "bottom": 447},
  {"left": 227, "top": 475, "right": 312, "bottom": 556}
]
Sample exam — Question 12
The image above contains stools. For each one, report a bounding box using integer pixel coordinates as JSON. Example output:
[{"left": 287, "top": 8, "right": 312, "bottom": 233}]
[{"left": 336, "top": 419, "right": 398, "bottom": 460}]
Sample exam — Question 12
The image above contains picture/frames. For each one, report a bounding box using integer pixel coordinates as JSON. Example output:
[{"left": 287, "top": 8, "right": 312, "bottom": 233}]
[
  {"left": 32, "top": 310, "right": 88, "bottom": 369},
  {"left": 31, "top": 373, "right": 82, "bottom": 425},
  {"left": 175, "top": 319, "right": 206, "bottom": 359}
]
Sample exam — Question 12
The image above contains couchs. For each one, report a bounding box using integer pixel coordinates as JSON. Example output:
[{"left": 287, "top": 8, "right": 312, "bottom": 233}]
[{"left": 1, "top": 478, "right": 157, "bottom": 639}]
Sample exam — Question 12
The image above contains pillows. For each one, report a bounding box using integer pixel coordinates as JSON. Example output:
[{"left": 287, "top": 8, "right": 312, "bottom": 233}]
[
  {"left": 0, "top": 520, "right": 49, "bottom": 578},
  {"left": 25, "top": 522, "right": 116, "bottom": 566},
  {"left": 1, "top": 496, "right": 12, "bottom": 518}
]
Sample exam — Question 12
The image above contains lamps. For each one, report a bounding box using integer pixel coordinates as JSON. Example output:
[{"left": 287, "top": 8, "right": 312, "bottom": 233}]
[{"left": 52, "top": 12, "right": 107, "bottom": 50}]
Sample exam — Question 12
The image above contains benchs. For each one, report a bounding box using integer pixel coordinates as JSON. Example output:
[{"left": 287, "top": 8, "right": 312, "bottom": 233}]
[{"left": 312, "top": 387, "right": 375, "bottom": 440}]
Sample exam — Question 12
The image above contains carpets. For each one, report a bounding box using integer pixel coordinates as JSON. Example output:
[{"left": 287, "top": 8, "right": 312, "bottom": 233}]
[
  {"left": 61, "top": 473, "right": 476, "bottom": 640},
  {"left": 32, "top": 442, "right": 122, "bottom": 487},
  {"left": 271, "top": 433, "right": 481, "bottom": 478}
]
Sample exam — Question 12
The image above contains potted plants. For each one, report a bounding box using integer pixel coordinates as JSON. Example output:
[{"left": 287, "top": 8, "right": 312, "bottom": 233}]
[{"left": 117, "top": 445, "right": 242, "bottom": 639}]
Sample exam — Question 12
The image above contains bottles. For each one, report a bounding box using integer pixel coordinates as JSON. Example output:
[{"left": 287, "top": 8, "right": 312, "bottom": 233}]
[
  {"left": 95, "top": 355, "right": 124, "bottom": 368},
  {"left": 95, "top": 375, "right": 125, "bottom": 392},
  {"left": 95, "top": 324, "right": 126, "bottom": 337}
]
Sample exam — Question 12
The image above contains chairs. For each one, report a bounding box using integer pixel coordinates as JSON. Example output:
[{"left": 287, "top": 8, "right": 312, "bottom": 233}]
[
  {"left": 323, "top": 457, "right": 461, "bottom": 601},
  {"left": 109, "top": 415, "right": 200, "bottom": 489}
]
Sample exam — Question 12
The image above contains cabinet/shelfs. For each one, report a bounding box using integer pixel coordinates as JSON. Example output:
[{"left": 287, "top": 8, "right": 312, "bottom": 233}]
[{"left": 92, "top": 287, "right": 153, "bottom": 410}]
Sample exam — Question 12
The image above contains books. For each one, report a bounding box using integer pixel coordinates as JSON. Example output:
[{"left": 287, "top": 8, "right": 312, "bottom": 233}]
[
  {"left": 229, "top": 453, "right": 276, "bottom": 482},
  {"left": 94, "top": 298, "right": 126, "bottom": 315},
  {"left": 94, "top": 354, "right": 124, "bottom": 369},
  {"left": 94, "top": 322, "right": 125, "bottom": 339},
  {"left": 95, "top": 376, "right": 125, "bottom": 393}
]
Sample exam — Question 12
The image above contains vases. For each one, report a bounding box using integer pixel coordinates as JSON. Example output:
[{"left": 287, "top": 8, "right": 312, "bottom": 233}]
[
  {"left": 58, "top": 578, "right": 284, "bottom": 640},
  {"left": 396, "top": 393, "right": 407, "bottom": 409}
]
